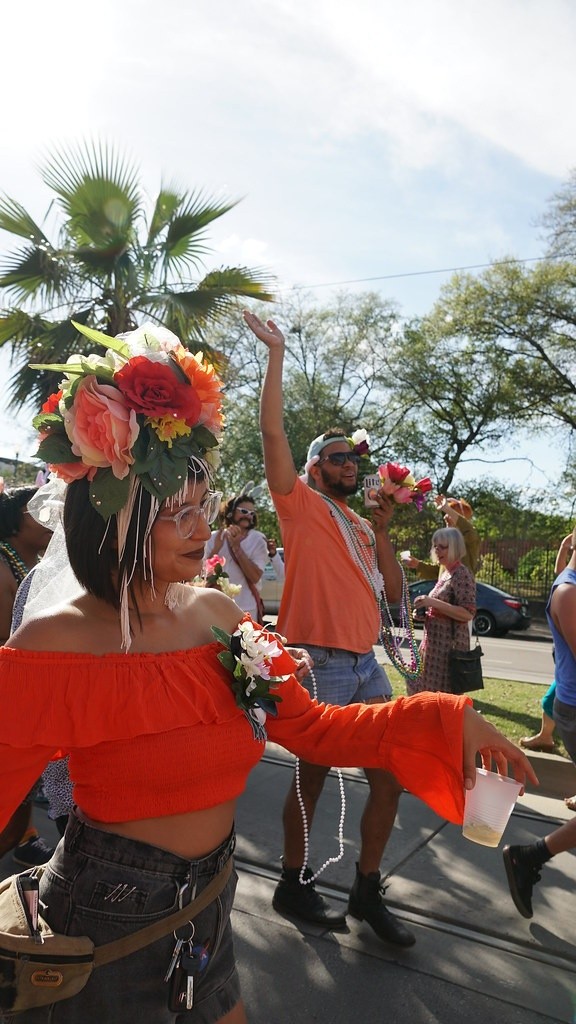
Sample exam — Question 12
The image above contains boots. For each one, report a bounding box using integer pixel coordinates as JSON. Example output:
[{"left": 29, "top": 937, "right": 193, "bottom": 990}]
[
  {"left": 272, "top": 853, "right": 346, "bottom": 929},
  {"left": 347, "top": 861, "right": 416, "bottom": 947}
]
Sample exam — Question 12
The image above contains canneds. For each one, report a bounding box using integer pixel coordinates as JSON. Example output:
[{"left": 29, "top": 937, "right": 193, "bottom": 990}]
[{"left": 416, "top": 606, "right": 425, "bottom": 616}]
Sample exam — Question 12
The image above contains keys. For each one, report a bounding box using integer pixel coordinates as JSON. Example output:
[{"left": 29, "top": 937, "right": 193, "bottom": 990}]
[{"left": 164, "top": 939, "right": 201, "bottom": 1013}]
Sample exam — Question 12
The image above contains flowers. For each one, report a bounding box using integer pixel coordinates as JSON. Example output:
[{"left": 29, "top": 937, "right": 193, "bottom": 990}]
[
  {"left": 208, "top": 621, "right": 292, "bottom": 727},
  {"left": 204, "top": 552, "right": 242, "bottom": 603},
  {"left": 28, "top": 319, "right": 226, "bottom": 523},
  {"left": 372, "top": 462, "right": 433, "bottom": 526},
  {"left": 345, "top": 428, "right": 372, "bottom": 460}
]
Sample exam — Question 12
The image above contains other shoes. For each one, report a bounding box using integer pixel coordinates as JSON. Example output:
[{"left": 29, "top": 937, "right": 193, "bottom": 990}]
[{"left": 520, "top": 736, "right": 555, "bottom": 749}]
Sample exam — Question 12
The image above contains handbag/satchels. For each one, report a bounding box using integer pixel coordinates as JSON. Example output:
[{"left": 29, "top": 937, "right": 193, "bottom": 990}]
[
  {"left": 256, "top": 595, "right": 266, "bottom": 627},
  {"left": 0, "top": 857, "right": 234, "bottom": 1017},
  {"left": 447, "top": 646, "right": 485, "bottom": 693}
]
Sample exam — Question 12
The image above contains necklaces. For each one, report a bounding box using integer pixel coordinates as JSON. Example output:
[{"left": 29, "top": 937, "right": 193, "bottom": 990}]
[
  {"left": 312, "top": 487, "right": 425, "bottom": 682},
  {"left": 0, "top": 542, "right": 40, "bottom": 587},
  {"left": 296, "top": 657, "right": 345, "bottom": 884}
]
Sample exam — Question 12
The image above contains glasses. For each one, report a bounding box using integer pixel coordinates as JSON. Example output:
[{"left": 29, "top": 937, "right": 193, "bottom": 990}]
[
  {"left": 236, "top": 506, "right": 256, "bottom": 518},
  {"left": 434, "top": 544, "right": 449, "bottom": 551},
  {"left": 315, "top": 452, "right": 361, "bottom": 467},
  {"left": 159, "top": 490, "right": 225, "bottom": 540}
]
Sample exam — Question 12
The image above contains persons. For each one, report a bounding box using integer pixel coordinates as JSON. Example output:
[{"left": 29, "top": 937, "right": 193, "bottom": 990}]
[
  {"left": 0, "top": 485, "right": 52, "bottom": 860},
  {"left": 244, "top": 309, "right": 420, "bottom": 953},
  {"left": 401, "top": 496, "right": 483, "bottom": 695},
  {"left": 505, "top": 527, "right": 576, "bottom": 918},
  {"left": 202, "top": 482, "right": 284, "bottom": 623},
  {"left": 13, "top": 562, "right": 315, "bottom": 865},
  {"left": 0, "top": 457, "right": 538, "bottom": 1024}
]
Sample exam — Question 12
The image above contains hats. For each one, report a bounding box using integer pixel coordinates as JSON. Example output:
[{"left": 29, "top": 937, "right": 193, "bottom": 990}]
[{"left": 305, "top": 430, "right": 353, "bottom": 490}]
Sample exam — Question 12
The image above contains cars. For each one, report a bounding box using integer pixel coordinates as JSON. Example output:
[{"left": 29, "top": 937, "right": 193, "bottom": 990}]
[
  {"left": 381, "top": 579, "right": 532, "bottom": 639},
  {"left": 258, "top": 547, "right": 288, "bottom": 615}
]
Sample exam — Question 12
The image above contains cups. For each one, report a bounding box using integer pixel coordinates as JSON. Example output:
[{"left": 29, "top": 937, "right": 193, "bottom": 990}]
[
  {"left": 461, "top": 767, "right": 523, "bottom": 848},
  {"left": 400, "top": 550, "right": 410, "bottom": 560}
]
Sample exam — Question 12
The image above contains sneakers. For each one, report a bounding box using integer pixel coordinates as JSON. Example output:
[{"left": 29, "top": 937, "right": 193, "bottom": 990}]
[{"left": 503, "top": 843, "right": 545, "bottom": 919}]
